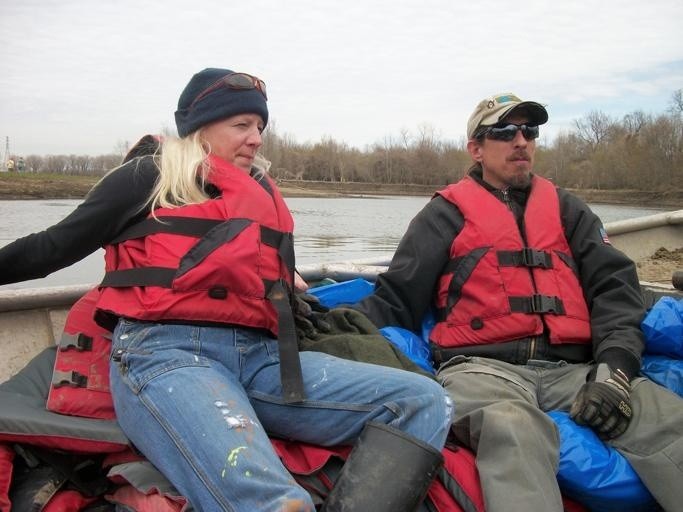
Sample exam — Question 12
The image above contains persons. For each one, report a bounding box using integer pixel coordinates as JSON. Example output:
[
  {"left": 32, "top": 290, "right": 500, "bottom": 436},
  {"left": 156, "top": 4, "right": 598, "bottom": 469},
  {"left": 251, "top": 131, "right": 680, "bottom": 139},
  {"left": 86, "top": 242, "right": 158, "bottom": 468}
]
[
  {"left": 0, "top": 67, "right": 454, "bottom": 512},
  {"left": 328, "top": 93, "right": 682, "bottom": 512}
]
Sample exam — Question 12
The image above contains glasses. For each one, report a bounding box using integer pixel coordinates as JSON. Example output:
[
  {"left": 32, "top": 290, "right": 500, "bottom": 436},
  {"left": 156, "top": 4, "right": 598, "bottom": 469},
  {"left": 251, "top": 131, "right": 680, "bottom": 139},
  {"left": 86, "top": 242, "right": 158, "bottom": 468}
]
[
  {"left": 469, "top": 121, "right": 542, "bottom": 141},
  {"left": 188, "top": 71, "right": 268, "bottom": 108}
]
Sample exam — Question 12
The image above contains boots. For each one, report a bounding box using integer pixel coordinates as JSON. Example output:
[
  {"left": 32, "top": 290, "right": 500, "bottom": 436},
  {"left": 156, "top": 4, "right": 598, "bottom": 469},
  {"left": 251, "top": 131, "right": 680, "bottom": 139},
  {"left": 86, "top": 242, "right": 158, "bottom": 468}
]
[{"left": 316, "top": 417, "right": 446, "bottom": 511}]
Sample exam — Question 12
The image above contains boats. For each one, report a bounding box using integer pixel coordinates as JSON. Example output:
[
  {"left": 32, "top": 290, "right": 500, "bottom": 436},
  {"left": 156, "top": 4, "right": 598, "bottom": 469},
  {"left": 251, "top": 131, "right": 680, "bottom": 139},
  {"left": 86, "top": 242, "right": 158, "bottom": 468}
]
[{"left": 0, "top": 209, "right": 682, "bottom": 512}]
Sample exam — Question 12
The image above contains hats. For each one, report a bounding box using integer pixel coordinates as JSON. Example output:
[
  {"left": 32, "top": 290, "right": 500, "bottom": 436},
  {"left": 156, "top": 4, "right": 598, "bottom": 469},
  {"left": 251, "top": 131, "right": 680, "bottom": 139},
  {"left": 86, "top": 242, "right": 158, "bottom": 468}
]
[
  {"left": 465, "top": 94, "right": 551, "bottom": 141},
  {"left": 172, "top": 66, "right": 269, "bottom": 137}
]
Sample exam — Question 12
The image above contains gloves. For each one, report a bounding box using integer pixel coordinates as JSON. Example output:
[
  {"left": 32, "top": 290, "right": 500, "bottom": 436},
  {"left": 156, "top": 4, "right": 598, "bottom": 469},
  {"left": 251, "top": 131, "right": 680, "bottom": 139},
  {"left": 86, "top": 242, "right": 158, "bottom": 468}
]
[
  {"left": 566, "top": 344, "right": 647, "bottom": 441},
  {"left": 289, "top": 286, "right": 338, "bottom": 343}
]
